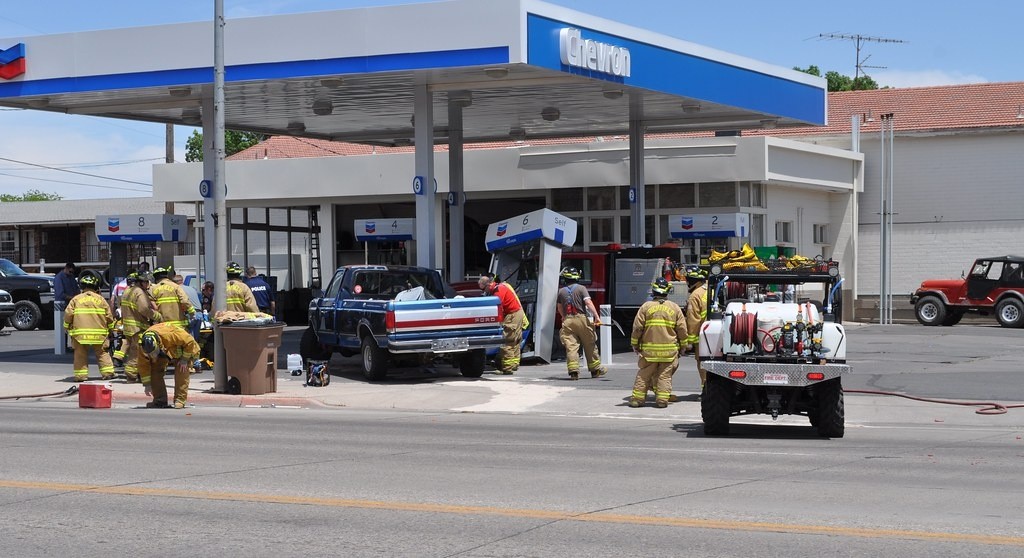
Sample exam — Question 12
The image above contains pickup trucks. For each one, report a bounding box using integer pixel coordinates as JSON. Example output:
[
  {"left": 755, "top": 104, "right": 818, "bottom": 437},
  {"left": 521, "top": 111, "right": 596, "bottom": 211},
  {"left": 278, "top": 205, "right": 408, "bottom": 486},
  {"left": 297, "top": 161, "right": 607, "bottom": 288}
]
[
  {"left": 300, "top": 265, "right": 503, "bottom": 380},
  {"left": 448, "top": 242, "right": 765, "bottom": 360}
]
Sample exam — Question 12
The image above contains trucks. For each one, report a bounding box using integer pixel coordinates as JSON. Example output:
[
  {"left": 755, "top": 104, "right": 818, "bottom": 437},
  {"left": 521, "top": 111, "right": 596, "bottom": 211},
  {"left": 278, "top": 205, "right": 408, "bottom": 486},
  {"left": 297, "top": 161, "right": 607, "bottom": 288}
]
[{"left": 699, "top": 260, "right": 851, "bottom": 439}]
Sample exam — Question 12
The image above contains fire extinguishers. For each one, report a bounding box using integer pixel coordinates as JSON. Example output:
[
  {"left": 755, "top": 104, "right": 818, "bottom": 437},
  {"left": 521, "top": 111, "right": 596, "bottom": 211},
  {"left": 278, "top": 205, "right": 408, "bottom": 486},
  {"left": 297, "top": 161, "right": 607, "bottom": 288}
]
[{"left": 665, "top": 257, "right": 672, "bottom": 281}]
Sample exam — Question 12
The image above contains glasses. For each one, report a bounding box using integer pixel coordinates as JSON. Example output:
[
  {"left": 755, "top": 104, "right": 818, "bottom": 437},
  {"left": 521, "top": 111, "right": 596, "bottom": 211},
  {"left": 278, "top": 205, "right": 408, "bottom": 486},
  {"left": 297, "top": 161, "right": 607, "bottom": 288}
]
[
  {"left": 69, "top": 271, "right": 74, "bottom": 273},
  {"left": 202, "top": 289, "right": 206, "bottom": 291},
  {"left": 481, "top": 285, "right": 487, "bottom": 290}
]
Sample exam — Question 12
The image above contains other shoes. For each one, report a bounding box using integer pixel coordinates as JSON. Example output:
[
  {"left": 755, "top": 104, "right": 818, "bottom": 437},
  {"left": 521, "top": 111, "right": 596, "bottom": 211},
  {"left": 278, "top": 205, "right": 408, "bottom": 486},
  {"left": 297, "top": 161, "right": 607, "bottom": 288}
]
[
  {"left": 571, "top": 372, "right": 579, "bottom": 380},
  {"left": 114, "top": 359, "right": 122, "bottom": 367},
  {"left": 146, "top": 401, "right": 168, "bottom": 407},
  {"left": 657, "top": 402, "right": 667, "bottom": 408},
  {"left": 628, "top": 400, "right": 639, "bottom": 408},
  {"left": 668, "top": 395, "right": 677, "bottom": 402},
  {"left": 174, "top": 401, "right": 183, "bottom": 409},
  {"left": 195, "top": 367, "right": 202, "bottom": 373},
  {"left": 591, "top": 366, "right": 608, "bottom": 378}
]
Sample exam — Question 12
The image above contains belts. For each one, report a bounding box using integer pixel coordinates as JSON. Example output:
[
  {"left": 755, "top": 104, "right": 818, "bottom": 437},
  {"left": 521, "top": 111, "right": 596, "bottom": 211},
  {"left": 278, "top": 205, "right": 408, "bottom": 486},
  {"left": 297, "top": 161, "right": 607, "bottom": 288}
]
[{"left": 185, "top": 309, "right": 201, "bottom": 314}]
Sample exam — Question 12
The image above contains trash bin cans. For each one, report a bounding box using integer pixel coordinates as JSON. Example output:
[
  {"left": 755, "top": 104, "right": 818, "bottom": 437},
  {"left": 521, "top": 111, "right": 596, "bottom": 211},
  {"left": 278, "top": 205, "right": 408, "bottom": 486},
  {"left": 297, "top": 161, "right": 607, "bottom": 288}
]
[{"left": 218, "top": 321, "right": 288, "bottom": 395}]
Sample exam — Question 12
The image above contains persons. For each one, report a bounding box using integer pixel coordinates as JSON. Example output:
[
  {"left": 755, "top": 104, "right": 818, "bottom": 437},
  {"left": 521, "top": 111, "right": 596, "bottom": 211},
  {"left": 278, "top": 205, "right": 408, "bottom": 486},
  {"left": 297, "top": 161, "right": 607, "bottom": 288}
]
[
  {"left": 478, "top": 273, "right": 529, "bottom": 376},
  {"left": 54, "top": 262, "right": 275, "bottom": 409},
  {"left": 631, "top": 266, "right": 718, "bottom": 407},
  {"left": 556, "top": 266, "right": 607, "bottom": 380}
]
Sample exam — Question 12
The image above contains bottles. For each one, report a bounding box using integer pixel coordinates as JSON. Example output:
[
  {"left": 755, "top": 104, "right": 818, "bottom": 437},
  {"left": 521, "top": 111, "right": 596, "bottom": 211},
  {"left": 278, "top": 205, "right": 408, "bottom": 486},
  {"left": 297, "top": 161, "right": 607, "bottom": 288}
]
[{"left": 287, "top": 352, "right": 303, "bottom": 373}]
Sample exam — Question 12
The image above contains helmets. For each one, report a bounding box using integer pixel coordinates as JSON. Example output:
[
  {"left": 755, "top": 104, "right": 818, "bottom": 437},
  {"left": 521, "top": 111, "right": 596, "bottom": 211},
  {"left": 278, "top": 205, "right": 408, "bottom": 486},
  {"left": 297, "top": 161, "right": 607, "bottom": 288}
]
[
  {"left": 153, "top": 266, "right": 169, "bottom": 276},
  {"left": 560, "top": 267, "right": 581, "bottom": 279},
  {"left": 80, "top": 275, "right": 99, "bottom": 290},
  {"left": 136, "top": 270, "right": 155, "bottom": 283},
  {"left": 684, "top": 265, "right": 708, "bottom": 279},
  {"left": 652, "top": 277, "right": 672, "bottom": 294},
  {"left": 126, "top": 273, "right": 138, "bottom": 286},
  {"left": 138, "top": 331, "right": 160, "bottom": 358},
  {"left": 226, "top": 262, "right": 243, "bottom": 275}
]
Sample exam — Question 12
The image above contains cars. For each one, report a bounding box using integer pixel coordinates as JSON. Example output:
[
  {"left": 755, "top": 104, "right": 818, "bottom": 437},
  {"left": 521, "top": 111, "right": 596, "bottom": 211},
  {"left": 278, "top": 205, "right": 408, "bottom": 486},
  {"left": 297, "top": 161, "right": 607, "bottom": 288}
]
[
  {"left": 184, "top": 275, "right": 206, "bottom": 305},
  {"left": 0, "top": 290, "right": 15, "bottom": 331}
]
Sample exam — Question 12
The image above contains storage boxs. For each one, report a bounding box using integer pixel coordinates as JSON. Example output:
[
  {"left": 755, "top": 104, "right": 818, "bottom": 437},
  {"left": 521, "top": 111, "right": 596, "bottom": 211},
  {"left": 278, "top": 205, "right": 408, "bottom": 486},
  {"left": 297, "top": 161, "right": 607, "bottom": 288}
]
[
  {"left": 78, "top": 381, "right": 113, "bottom": 408},
  {"left": 615, "top": 258, "right": 689, "bottom": 307},
  {"left": 394, "top": 286, "right": 426, "bottom": 301}
]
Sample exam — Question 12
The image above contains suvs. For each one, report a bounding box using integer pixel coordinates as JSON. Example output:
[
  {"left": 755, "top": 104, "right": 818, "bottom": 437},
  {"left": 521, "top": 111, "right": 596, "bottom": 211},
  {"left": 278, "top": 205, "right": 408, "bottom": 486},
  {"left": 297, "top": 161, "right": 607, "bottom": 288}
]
[
  {"left": 909, "top": 253, "right": 1024, "bottom": 327},
  {"left": 0, "top": 258, "right": 55, "bottom": 331}
]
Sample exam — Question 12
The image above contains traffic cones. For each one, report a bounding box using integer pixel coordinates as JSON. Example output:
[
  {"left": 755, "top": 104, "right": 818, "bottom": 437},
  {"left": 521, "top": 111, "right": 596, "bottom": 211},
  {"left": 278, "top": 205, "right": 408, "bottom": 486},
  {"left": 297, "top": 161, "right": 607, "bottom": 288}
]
[{"left": 664, "top": 260, "right": 671, "bottom": 281}]
